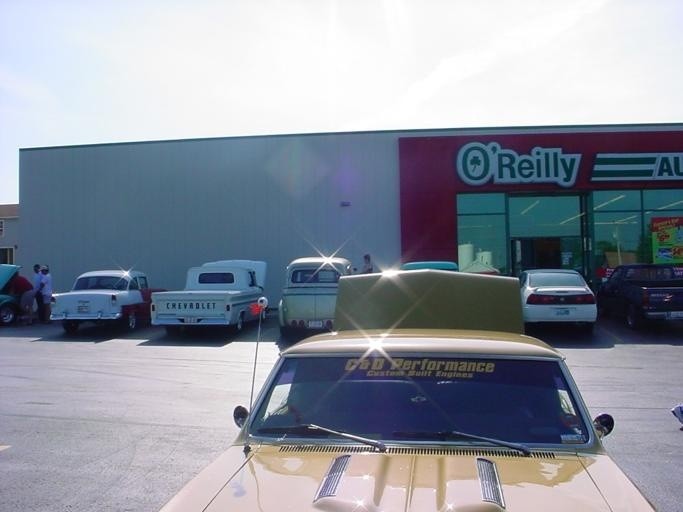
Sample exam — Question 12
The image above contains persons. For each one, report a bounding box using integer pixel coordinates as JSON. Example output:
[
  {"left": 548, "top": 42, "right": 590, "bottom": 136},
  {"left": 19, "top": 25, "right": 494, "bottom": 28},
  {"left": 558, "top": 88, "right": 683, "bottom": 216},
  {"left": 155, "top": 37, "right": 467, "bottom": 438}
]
[
  {"left": 32, "top": 263, "right": 45, "bottom": 321},
  {"left": 592, "top": 260, "right": 610, "bottom": 294},
  {"left": 37, "top": 265, "right": 52, "bottom": 324},
  {"left": 357, "top": 253, "right": 373, "bottom": 274}
]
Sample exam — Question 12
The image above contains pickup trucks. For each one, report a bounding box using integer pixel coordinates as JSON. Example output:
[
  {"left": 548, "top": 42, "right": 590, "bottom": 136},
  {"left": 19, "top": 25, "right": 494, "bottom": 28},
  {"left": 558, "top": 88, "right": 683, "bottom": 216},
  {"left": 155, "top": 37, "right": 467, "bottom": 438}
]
[
  {"left": 594, "top": 262, "right": 682, "bottom": 335},
  {"left": 150, "top": 260, "right": 266, "bottom": 336}
]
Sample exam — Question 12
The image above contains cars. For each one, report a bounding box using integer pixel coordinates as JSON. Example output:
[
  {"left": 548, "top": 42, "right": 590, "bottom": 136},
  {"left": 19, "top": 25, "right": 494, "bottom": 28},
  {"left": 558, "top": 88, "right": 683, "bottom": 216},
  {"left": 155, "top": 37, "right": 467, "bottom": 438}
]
[
  {"left": 0, "top": 264, "right": 24, "bottom": 326},
  {"left": 518, "top": 269, "right": 598, "bottom": 338},
  {"left": 49, "top": 270, "right": 167, "bottom": 333},
  {"left": 155, "top": 269, "right": 658, "bottom": 512},
  {"left": 400, "top": 262, "right": 458, "bottom": 272},
  {"left": 278, "top": 257, "right": 355, "bottom": 341}
]
